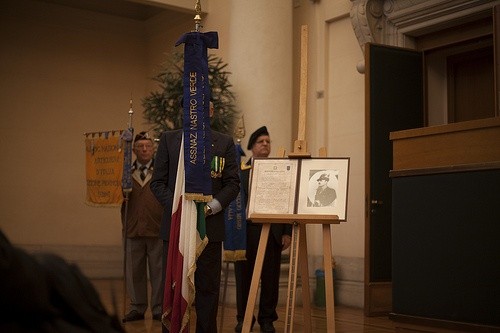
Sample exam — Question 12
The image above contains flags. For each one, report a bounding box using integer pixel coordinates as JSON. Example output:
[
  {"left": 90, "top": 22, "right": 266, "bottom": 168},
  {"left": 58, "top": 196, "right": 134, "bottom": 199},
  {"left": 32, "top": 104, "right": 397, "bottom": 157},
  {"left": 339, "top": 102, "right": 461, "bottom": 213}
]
[{"left": 163, "top": 131, "right": 208, "bottom": 333}]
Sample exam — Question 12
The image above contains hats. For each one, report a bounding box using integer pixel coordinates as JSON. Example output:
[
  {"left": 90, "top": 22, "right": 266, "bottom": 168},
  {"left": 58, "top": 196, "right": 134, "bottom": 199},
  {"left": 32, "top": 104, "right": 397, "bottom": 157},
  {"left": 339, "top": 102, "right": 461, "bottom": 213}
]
[
  {"left": 134, "top": 130, "right": 154, "bottom": 143},
  {"left": 247, "top": 126, "right": 269, "bottom": 151}
]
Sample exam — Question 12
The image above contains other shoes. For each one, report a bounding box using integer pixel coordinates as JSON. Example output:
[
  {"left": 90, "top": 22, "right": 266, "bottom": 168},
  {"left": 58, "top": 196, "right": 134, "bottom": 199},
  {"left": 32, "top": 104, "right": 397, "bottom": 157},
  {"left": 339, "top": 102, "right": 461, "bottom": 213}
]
[
  {"left": 234, "top": 315, "right": 256, "bottom": 333},
  {"left": 152, "top": 306, "right": 164, "bottom": 321},
  {"left": 260, "top": 321, "right": 275, "bottom": 333},
  {"left": 122, "top": 309, "right": 144, "bottom": 323}
]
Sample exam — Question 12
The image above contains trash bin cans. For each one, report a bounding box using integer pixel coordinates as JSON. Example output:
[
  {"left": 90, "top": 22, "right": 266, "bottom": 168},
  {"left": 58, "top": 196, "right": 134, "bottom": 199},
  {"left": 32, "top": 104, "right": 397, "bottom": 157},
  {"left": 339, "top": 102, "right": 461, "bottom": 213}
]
[{"left": 314, "top": 269, "right": 338, "bottom": 308}]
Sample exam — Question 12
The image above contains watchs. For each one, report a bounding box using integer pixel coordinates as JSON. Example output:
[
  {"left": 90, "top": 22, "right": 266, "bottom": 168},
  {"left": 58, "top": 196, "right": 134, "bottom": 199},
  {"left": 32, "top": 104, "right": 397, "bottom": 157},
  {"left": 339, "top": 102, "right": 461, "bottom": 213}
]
[{"left": 206, "top": 205, "right": 212, "bottom": 215}]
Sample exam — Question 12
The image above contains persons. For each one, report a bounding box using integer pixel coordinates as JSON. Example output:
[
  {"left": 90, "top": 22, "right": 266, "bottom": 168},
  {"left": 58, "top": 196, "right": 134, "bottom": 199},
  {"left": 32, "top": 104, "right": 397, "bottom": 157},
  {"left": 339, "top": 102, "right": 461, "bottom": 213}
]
[
  {"left": 313, "top": 173, "right": 336, "bottom": 207},
  {"left": 0, "top": 231, "right": 130, "bottom": 333},
  {"left": 234, "top": 126, "right": 292, "bottom": 333},
  {"left": 122, "top": 132, "right": 162, "bottom": 322},
  {"left": 151, "top": 99, "right": 241, "bottom": 333}
]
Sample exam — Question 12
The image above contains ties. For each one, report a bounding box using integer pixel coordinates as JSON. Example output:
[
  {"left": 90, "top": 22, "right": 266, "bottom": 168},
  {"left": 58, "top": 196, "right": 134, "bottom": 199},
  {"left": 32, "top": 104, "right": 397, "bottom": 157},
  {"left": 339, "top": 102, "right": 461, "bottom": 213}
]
[{"left": 139, "top": 165, "right": 146, "bottom": 182}]
